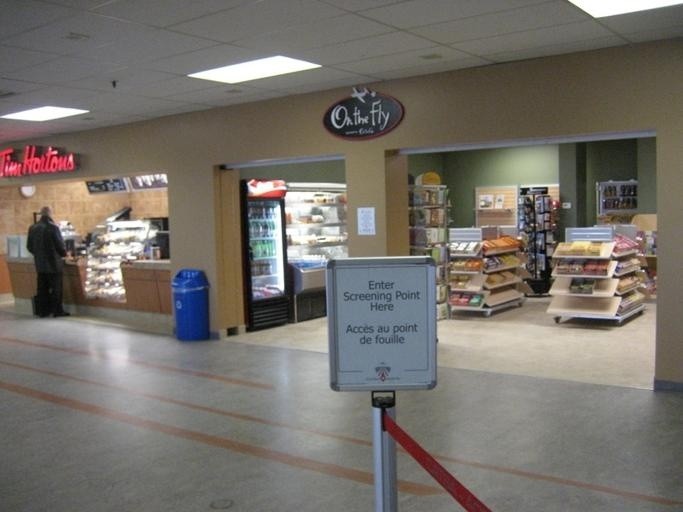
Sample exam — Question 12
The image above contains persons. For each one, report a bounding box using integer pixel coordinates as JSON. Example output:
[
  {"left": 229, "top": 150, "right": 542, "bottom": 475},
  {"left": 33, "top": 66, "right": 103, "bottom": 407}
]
[{"left": 26, "top": 204, "right": 72, "bottom": 319}]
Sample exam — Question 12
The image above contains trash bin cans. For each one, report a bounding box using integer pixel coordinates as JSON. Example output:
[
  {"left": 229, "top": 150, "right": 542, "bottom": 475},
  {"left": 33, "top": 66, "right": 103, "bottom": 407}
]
[{"left": 171, "top": 269, "right": 210, "bottom": 341}]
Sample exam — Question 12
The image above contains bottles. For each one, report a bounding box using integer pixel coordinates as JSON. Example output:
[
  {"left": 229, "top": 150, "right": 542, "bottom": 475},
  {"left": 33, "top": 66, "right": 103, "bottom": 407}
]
[
  {"left": 246, "top": 205, "right": 277, "bottom": 276},
  {"left": 150, "top": 243, "right": 161, "bottom": 260}
]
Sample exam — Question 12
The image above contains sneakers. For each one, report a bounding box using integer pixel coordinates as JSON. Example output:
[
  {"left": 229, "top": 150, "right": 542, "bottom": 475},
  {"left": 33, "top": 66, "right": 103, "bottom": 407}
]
[{"left": 54, "top": 312, "right": 70, "bottom": 316}]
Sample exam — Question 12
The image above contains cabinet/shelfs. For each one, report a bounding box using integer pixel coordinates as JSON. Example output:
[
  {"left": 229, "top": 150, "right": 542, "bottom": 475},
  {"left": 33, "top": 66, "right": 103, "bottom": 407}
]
[
  {"left": 407, "top": 184, "right": 452, "bottom": 320},
  {"left": 446, "top": 241, "right": 646, "bottom": 327},
  {"left": 285, "top": 180, "right": 350, "bottom": 265}
]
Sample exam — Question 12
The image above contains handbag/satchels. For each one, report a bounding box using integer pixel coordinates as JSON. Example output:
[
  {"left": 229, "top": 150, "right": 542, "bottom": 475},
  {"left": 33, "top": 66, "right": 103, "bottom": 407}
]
[{"left": 32, "top": 294, "right": 53, "bottom": 314}]
